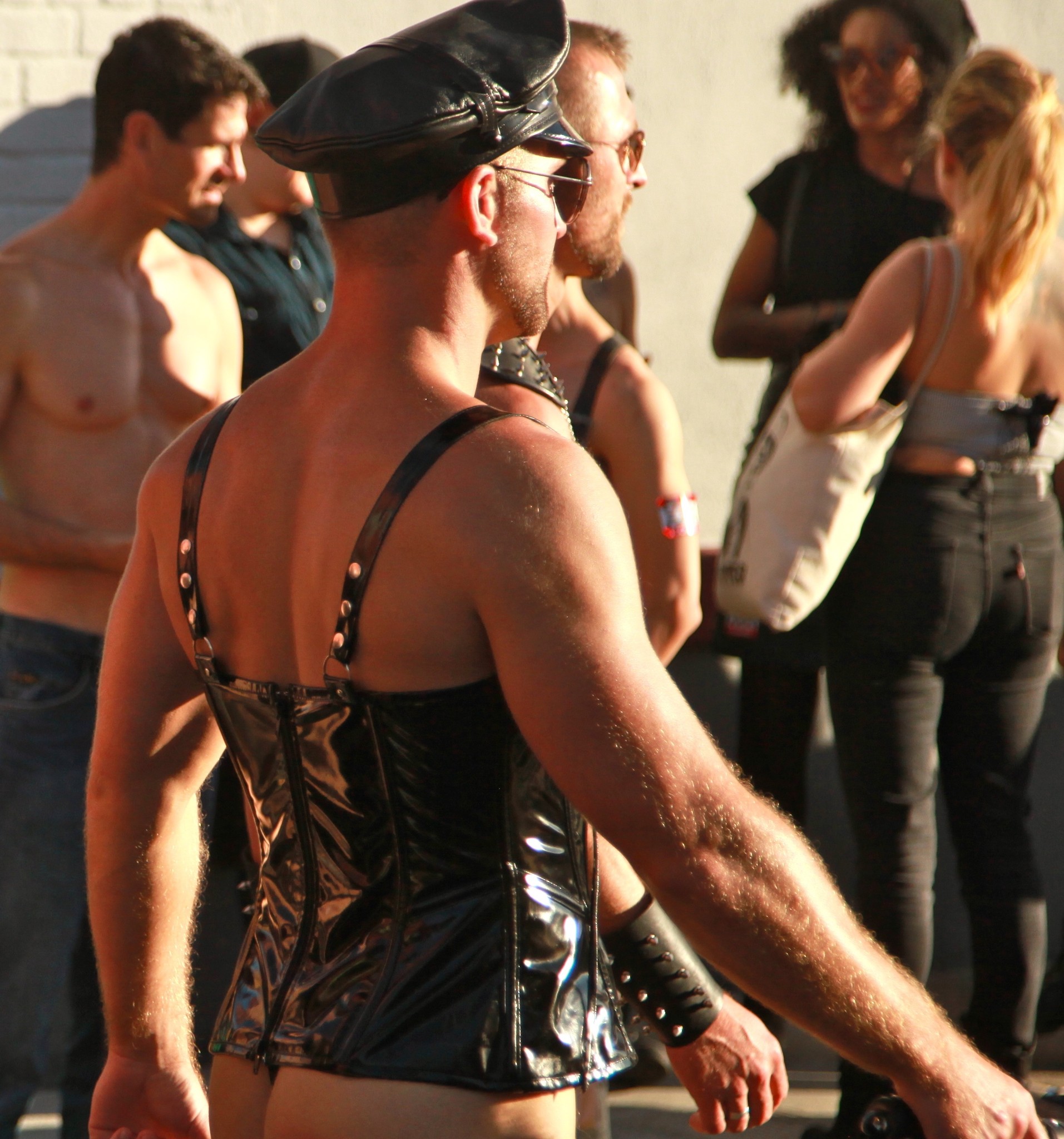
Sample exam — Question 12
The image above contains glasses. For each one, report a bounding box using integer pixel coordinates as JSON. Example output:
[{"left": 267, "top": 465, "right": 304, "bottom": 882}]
[{"left": 489, "top": 153, "right": 593, "bottom": 224}]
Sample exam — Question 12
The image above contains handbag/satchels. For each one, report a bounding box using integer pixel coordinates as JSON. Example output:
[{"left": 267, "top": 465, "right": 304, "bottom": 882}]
[{"left": 716, "top": 383, "right": 905, "bottom": 630}]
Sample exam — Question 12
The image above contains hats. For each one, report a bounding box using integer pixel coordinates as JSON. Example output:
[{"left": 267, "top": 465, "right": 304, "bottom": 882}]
[{"left": 255, "top": 0, "right": 593, "bottom": 219}]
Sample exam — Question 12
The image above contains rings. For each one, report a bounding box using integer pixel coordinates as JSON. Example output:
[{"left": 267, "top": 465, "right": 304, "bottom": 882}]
[{"left": 727, "top": 1105, "right": 749, "bottom": 1119}]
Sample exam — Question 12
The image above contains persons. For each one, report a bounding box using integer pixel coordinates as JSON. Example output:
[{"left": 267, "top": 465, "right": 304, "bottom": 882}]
[{"left": 0, "top": 0, "right": 1063, "bottom": 1139}]
